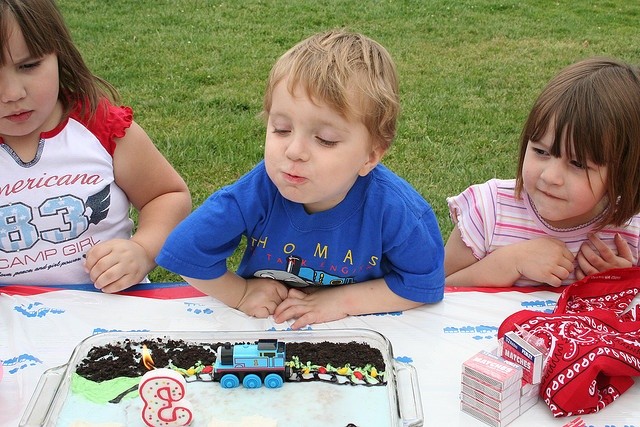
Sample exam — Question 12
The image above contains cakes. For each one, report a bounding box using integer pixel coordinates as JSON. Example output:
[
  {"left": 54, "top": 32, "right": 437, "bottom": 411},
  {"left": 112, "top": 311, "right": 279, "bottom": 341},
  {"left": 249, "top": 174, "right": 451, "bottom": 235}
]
[{"left": 57, "top": 341, "right": 389, "bottom": 426}]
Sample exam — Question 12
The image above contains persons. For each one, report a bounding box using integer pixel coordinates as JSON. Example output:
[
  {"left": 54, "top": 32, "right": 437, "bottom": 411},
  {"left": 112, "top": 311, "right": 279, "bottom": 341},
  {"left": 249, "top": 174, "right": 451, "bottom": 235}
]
[
  {"left": 444, "top": 58, "right": 635, "bottom": 287},
  {"left": 1, "top": 1, "right": 193, "bottom": 295},
  {"left": 155, "top": 26, "right": 445, "bottom": 330}
]
